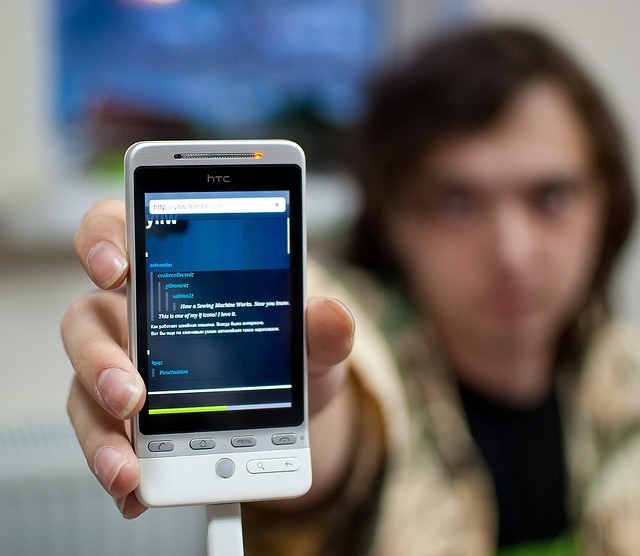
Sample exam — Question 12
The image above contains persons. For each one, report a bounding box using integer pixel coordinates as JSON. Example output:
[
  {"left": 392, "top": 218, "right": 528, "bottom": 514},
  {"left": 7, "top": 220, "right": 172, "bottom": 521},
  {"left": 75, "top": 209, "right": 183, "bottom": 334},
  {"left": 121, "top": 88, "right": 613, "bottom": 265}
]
[{"left": 58, "top": 21, "right": 640, "bottom": 555}]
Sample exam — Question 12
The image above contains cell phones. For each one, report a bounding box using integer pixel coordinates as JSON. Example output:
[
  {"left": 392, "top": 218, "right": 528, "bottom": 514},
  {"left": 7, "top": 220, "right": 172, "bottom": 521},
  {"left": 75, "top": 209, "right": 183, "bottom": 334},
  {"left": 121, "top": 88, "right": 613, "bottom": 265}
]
[{"left": 124, "top": 139, "right": 312, "bottom": 506}]
